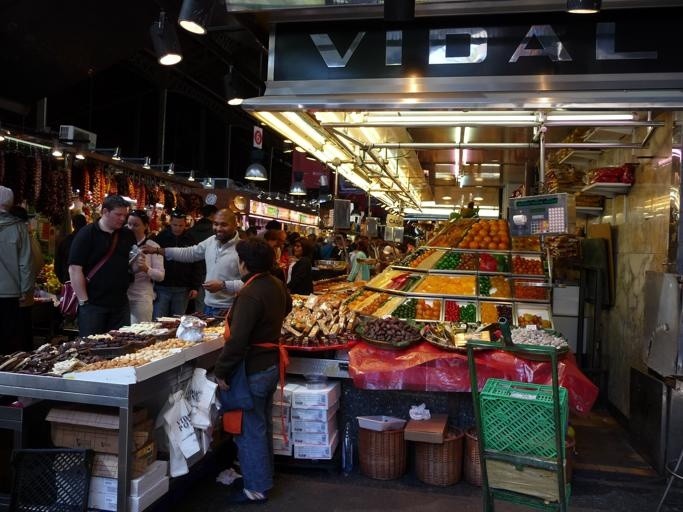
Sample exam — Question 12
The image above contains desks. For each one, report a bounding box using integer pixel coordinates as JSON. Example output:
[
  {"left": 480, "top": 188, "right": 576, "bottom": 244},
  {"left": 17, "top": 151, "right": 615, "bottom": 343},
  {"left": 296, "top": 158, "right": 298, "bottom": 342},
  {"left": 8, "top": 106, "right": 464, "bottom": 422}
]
[
  {"left": 270, "top": 346, "right": 583, "bottom": 407},
  {"left": 0, "top": 335, "right": 231, "bottom": 511}
]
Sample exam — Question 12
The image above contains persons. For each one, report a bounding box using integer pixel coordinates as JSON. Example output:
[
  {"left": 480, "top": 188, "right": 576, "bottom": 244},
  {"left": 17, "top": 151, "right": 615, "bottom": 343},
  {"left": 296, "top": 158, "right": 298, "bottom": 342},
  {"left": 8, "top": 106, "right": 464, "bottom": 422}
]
[
  {"left": 214, "top": 237, "right": 293, "bottom": 503},
  {"left": 1, "top": 186, "right": 370, "bottom": 357}
]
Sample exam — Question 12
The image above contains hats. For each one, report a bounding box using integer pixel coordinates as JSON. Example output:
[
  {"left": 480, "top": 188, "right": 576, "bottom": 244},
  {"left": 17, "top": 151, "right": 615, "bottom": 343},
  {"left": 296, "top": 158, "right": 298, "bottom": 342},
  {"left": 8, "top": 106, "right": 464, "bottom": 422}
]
[
  {"left": 0, "top": 185, "right": 14, "bottom": 206},
  {"left": 9, "top": 206, "right": 36, "bottom": 222}
]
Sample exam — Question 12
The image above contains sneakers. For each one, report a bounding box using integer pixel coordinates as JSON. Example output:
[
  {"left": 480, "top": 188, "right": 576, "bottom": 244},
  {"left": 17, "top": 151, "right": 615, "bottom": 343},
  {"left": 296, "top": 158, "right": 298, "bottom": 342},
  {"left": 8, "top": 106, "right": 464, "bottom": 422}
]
[{"left": 226, "top": 484, "right": 273, "bottom": 505}]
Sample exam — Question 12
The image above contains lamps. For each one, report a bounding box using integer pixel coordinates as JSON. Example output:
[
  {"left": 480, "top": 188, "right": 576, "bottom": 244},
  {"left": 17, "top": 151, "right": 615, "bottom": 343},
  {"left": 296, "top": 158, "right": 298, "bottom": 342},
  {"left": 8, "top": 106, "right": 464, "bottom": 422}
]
[
  {"left": 357, "top": 107, "right": 640, "bottom": 126},
  {"left": 37, "top": 133, "right": 201, "bottom": 185},
  {"left": 236, "top": 110, "right": 333, "bottom": 217},
  {"left": 146, "top": 11, "right": 186, "bottom": 68},
  {"left": 173, "top": 0, "right": 216, "bottom": 38}
]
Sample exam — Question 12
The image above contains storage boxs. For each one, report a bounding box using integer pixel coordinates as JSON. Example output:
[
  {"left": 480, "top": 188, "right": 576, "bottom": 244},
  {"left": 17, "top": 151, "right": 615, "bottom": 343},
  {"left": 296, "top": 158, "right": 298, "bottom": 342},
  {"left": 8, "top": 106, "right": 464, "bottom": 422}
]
[
  {"left": 478, "top": 445, "right": 573, "bottom": 505},
  {"left": 270, "top": 375, "right": 342, "bottom": 462},
  {"left": 551, "top": 284, "right": 581, "bottom": 318},
  {"left": 39, "top": 402, "right": 176, "bottom": 512}
]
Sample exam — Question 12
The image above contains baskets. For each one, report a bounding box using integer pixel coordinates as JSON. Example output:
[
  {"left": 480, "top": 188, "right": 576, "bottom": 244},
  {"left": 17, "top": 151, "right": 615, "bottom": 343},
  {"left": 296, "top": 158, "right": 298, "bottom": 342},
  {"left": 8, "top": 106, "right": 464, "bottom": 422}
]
[
  {"left": 416, "top": 425, "right": 465, "bottom": 488},
  {"left": 357, "top": 426, "right": 408, "bottom": 480},
  {"left": 479, "top": 377, "right": 576, "bottom": 511},
  {"left": 464, "top": 426, "right": 485, "bottom": 486}
]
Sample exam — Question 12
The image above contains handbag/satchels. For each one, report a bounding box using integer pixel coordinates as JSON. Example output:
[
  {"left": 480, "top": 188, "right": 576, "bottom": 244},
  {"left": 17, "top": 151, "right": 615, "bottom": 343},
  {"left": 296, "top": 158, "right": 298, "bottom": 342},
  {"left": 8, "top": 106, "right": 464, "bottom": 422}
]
[
  {"left": 59, "top": 280, "right": 78, "bottom": 318},
  {"left": 30, "top": 238, "right": 44, "bottom": 276}
]
